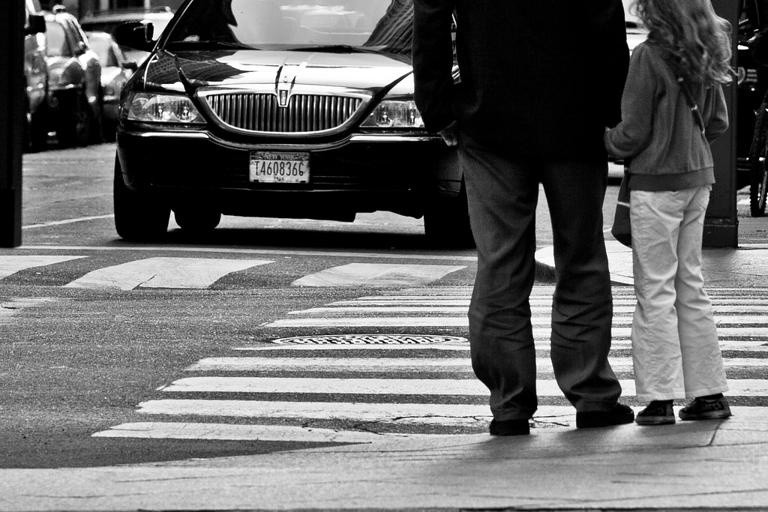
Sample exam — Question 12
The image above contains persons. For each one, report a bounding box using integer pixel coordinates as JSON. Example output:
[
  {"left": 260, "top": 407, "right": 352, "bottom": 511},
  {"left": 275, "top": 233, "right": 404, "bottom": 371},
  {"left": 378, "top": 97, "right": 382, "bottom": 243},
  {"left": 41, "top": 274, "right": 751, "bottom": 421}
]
[
  {"left": 604, "top": 0, "right": 739, "bottom": 425},
  {"left": 412, "top": 0, "right": 635, "bottom": 436}
]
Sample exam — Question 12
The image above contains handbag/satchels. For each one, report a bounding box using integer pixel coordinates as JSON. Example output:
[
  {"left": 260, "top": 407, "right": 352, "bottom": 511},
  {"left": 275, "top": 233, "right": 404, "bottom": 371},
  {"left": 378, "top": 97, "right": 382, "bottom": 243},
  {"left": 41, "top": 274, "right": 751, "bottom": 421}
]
[{"left": 612, "top": 173, "right": 707, "bottom": 248}]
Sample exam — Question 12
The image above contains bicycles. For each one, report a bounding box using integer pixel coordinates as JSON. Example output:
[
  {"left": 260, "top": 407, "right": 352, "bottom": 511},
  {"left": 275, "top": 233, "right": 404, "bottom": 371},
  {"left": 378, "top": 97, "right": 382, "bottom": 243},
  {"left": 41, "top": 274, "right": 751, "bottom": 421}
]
[{"left": 736, "top": 100, "right": 768, "bottom": 218}]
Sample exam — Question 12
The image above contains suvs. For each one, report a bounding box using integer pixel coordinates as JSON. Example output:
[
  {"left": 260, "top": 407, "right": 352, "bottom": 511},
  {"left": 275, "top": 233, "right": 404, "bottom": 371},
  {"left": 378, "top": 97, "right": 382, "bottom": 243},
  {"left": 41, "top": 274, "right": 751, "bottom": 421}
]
[{"left": 79, "top": 7, "right": 175, "bottom": 73}]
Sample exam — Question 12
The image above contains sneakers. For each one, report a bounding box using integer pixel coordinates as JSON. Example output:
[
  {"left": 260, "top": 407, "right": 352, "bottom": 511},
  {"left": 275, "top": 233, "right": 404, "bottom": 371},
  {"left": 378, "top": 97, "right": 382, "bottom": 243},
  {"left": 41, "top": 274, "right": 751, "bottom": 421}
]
[
  {"left": 679, "top": 396, "right": 732, "bottom": 420},
  {"left": 637, "top": 399, "right": 676, "bottom": 425}
]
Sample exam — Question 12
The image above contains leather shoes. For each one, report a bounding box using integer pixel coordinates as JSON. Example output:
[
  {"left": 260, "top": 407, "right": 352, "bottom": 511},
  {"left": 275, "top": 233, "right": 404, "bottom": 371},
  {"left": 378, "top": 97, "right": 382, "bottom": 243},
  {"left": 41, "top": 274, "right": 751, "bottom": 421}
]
[
  {"left": 576, "top": 403, "right": 634, "bottom": 429},
  {"left": 489, "top": 417, "right": 532, "bottom": 435}
]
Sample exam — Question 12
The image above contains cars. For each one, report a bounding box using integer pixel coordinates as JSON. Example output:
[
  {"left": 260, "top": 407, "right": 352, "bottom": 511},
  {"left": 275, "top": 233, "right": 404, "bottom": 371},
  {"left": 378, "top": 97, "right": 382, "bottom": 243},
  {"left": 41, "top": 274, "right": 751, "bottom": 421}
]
[
  {"left": 80, "top": 31, "right": 134, "bottom": 143},
  {"left": 113, "top": 1, "right": 477, "bottom": 252},
  {"left": 607, "top": 0, "right": 651, "bottom": 187},
  {"left": 20, "top": 1, "right": 49, "bottom": 153},
  {"left": 41, "top": 4, "right": 107, "bottom": 151},
  {"left": 279, "top": 5, "right": 373, "bottom": 48},
  {"left": 737, "top": 0, "right": 767, "bottom": 172}
]
